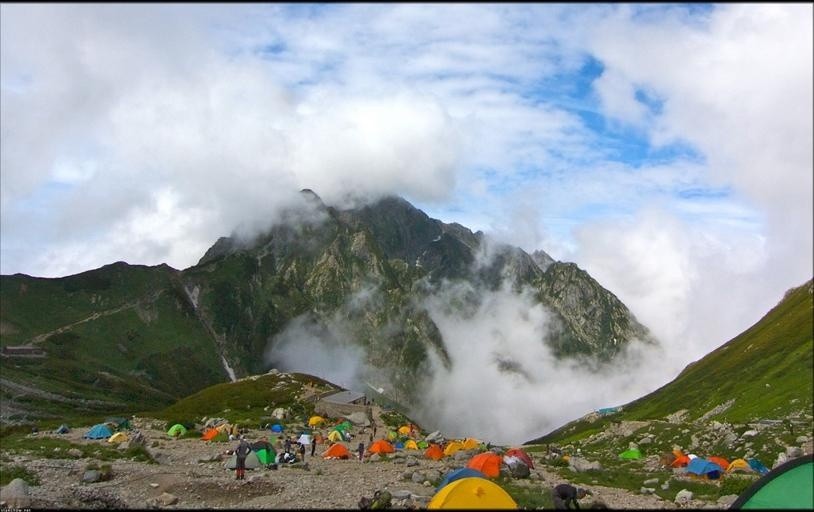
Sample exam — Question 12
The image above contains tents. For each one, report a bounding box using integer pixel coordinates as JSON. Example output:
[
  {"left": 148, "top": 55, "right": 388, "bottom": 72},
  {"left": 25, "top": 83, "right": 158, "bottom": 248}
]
[
  {"left": 225, "top": 440, "right": 277, "bottom": 470},
  {"left": 729, "top": 453, "right": 814, "bottom": 511},
  {"left": 167, "top": 424, "right": 239, "bottom": 442},
  {"left": 297, "top": 412, "right": 374, "bottom": 461},
  {"left": 427, "top": 468, "right": 520, "bottom": 511},
  {"left": 271, "top": 408, "right": 295, "bottom": 434},
  {"left": 369, "top": 425, "right": 573, "bottom": 480},
  {"left": 619, "top": 427, "right": 769, "bottom": 479},
  {"left": 83, "top": 419, "right": 128, "bottom": 443}
]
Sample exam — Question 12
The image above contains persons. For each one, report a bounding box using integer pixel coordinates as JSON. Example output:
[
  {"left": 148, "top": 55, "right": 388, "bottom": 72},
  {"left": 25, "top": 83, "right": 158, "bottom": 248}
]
[
  {"left": 370, "top": 492, "right": 393, "bottom": 511},
  {"left": 552, "top": 483, "right": 585, "bottom": 511},
  {"left": 233, "top": 435, "right": 251, "bottom": 480},
  {"left": 372, "top": 421, "right": 377, "bottom": 437},
  {"left": 276, "top": 433, "right": 316, "bottom": 464}
]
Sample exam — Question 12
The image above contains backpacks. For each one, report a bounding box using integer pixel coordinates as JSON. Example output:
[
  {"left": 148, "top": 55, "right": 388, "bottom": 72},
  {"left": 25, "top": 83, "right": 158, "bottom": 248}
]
[{"left": 367, "top": 490, "right": 392, "bottom": 510}]
